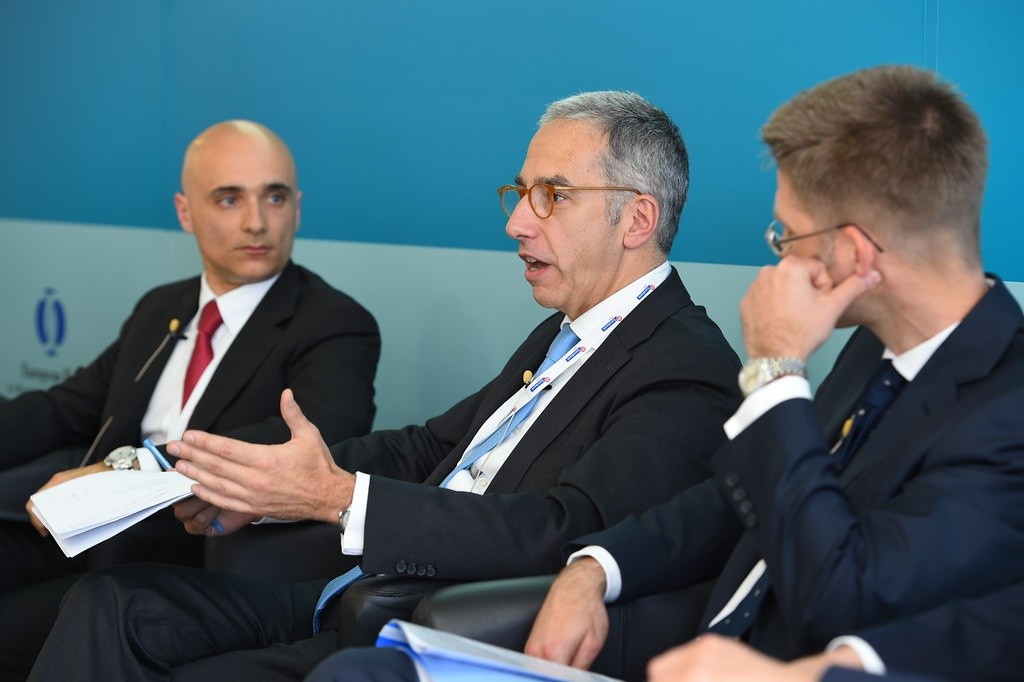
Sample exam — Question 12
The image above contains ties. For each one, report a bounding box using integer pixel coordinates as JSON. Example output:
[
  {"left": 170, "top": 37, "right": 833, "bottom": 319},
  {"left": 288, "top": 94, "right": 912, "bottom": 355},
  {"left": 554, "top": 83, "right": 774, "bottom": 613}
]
[
  {"left": 709, "top": 357, "right": 908, "bottom": 644},
  {"left": 181, "top": 299, "right": 224, "bottom": 411},
  {"left": 312, "top": 322, "right": 579, "bottom": 636}
]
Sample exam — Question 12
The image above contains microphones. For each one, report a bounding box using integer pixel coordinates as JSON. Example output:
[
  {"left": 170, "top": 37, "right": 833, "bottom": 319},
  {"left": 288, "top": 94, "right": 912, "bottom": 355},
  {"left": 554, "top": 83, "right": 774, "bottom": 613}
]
[
  {"left": 522, "top": 370, "right": 533, "bottom": 385},
  {"left": 841, "top": 419, "right": 853, "bottom": 439},
  {"left": 169, "top": 319, "right": 180, "bottom": 332}
]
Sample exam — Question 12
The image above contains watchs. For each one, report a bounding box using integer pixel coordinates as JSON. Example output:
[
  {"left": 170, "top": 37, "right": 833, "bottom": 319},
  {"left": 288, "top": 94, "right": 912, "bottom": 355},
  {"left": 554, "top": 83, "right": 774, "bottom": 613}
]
[
  {"left": 104, "top": 445, "right": 139, "bottom": 471},
  {"left": 738, "top": 356, "right": 808, "bottom": 398},
  {"left": 338, "top": 502, "right": 352, "bottom": 535}
]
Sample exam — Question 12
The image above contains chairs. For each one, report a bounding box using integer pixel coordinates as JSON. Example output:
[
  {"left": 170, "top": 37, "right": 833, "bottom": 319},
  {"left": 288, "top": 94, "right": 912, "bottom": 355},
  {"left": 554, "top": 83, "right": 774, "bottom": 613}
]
[{"left": 0, "top": 447, "right": 719, "bottom": 682}]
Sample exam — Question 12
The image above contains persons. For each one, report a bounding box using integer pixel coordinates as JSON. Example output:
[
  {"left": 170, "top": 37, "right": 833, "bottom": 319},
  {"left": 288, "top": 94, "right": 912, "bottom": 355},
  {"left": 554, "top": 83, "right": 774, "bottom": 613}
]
[
  {"left": 306, "top": 66, "right": 1024, "bottom": 682},
  {"left": 646, "top": 582, "right": 1024, "bottom": 682},
  {"left": 27, "top": 90, "right": 743, "bottom": 682},
  {"left": 0, "top": 121, "right": 381, "bottom": 682}
]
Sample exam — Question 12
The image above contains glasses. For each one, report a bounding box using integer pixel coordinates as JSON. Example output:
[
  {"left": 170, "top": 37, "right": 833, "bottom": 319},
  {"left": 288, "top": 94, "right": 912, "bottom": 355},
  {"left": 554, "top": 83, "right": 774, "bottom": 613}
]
[
  {"left": 500, "top": 184, "right": 642, "bottom": 219},
  {"left": 766, "top": 219, "right": 887, "bottom": 258}
]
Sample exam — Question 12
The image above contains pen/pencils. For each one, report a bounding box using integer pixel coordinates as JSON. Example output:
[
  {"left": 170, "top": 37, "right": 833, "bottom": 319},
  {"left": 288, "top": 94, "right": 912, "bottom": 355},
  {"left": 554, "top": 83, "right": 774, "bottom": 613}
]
[{"left": 143, "top": 437, "right": 225, "bottom": 535}]
